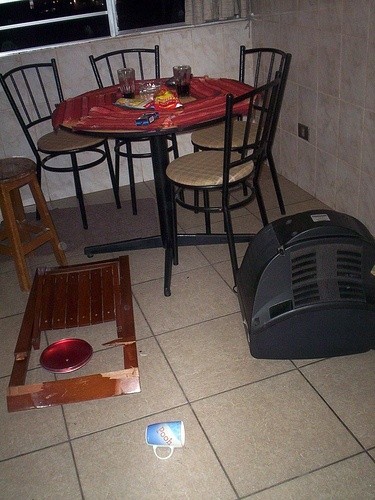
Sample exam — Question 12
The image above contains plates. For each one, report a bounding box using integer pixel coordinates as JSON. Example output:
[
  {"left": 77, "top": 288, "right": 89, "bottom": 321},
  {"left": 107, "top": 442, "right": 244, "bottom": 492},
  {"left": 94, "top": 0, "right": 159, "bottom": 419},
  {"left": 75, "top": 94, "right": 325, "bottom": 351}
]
[{"left": 39, "top": 337, "right": 93, "bottom": 373}]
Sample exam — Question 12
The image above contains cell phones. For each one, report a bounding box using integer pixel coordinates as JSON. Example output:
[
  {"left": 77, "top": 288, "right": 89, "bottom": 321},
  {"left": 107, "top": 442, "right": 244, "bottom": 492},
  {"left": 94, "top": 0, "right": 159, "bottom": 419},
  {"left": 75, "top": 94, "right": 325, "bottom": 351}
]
[{"left": 166, "top": 73, "right": 193, "bottom": 86}]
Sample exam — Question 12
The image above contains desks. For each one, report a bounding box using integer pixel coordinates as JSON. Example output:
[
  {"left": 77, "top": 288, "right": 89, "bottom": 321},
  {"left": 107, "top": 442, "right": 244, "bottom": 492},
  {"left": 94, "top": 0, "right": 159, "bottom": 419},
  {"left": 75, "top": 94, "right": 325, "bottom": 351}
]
[{"left": 48, "top": 73, "right": 262, "bottom": 297}]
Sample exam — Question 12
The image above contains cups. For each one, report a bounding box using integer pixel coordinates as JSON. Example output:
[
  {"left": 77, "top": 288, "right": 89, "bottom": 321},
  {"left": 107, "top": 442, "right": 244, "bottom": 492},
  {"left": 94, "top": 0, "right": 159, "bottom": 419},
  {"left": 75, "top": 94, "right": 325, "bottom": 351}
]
[
  {"left": 145, "top": 421, "right": 185, "bottom": 459},
  {"left": 173, "top": 65, "right": 191, "bottom": 98},
  {"left": 117, "top": 67, "right": 135, "bottom": 98}
]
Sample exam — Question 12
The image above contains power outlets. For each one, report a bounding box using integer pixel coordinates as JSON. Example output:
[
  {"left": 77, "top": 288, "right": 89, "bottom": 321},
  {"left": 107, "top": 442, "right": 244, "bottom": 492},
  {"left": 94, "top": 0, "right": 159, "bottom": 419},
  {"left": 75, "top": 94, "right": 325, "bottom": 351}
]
[{"left": 298, "top": 123, "right": 310, "bottom": 141}]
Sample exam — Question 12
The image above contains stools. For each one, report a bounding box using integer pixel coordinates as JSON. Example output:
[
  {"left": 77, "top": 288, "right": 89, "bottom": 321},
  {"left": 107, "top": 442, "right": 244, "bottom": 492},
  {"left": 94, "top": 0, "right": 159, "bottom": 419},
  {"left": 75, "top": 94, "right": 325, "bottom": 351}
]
[{"left": 0, "top": 157, "right": 68, "bottom": 291}]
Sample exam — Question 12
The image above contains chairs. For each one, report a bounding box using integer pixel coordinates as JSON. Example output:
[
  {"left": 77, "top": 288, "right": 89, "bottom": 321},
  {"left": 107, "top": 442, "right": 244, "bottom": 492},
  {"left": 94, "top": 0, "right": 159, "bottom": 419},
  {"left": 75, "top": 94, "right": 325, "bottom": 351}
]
[
  {"left": 192, "top": 42, "right": 296, "bottom": 215},
  {"left": 0, "top": 58, "right": 124, "bottom": 232},
  {"left": 86, "top": 43, "right": 181, "bottom": 215},
  {"left": 172, "top": 70, "right": 284, "bottom": 289}
]
